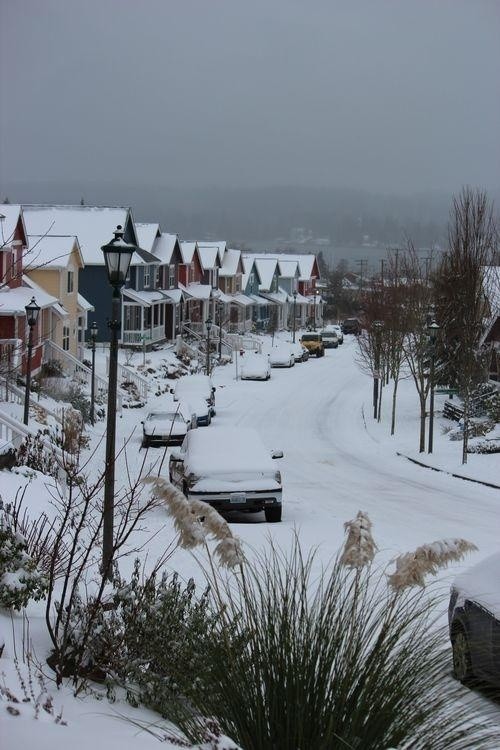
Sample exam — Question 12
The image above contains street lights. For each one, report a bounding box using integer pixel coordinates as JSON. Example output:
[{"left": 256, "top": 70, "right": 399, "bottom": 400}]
[
  {"left": 314, "top": 289, "right": 320, "bottom": 332},
  {"left": 218, "top": 300, "right": 225, "bottom": 359},
  {"left": 292, "top": 290, "right": 298, "bottom": 343},
  {"left": 89, "top": 321, "right": 100, "bottom": 426},
  {"left": 91, "top": 225, "right": 138, "bottom": 611},
  {"left": 374, "top": 321, "right": 385, "bottom": 418},
  {"left": 428, "top": 319, "right": 440, "bottom": 455},
  {"left": 204, "top": 315, "right": 213, "bottom": 376},
  {"left": 270, "top": 307, "right": 277, "bottom": 347},
  {"left": 23, "top": 295, "right": 41, "bottom": 426}
]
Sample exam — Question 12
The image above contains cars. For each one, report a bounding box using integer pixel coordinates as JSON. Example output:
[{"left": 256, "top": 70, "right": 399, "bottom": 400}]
[
  {"left": 267, "top": 346, "right": 294, "bottom": 368},
  {"left": 287, "top": 343, "right": 310, "bottom": 363},
  {"left": 140, "top": 405, "right": 190, "bottom": 448},
  {"left": 240, "top": 354, "right": 271, "bottom": 381},
  {"left": 448, "top": 547, "right": 500, "bottom": 691},
  {"left": 169, "top": 425, "right": 284, "bottom": 523},
  {"left": 170, "top": 375, "right": 216, "bottom": 406},
  {"left": 175, "top": 395, "right": 212, "bottom": 426}
]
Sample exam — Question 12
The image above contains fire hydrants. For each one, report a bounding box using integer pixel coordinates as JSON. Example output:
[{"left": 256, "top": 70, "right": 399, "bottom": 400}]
[{"left": 239, "top": 347, "right": 245, "bottom": 357}]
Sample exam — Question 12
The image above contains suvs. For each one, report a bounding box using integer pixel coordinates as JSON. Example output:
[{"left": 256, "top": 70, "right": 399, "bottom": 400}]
[
  {"left": 340, "top": 318, "right": 362, "bottom": 335},
  {"left": 326, "top": 325, "right": 344, "bottom": 344},
  {"left": 298, "top": 333, "right": 324, "bottom": 358},
  {"left": 320, "top": 328, "right": 339, "bottom": 348}
]
